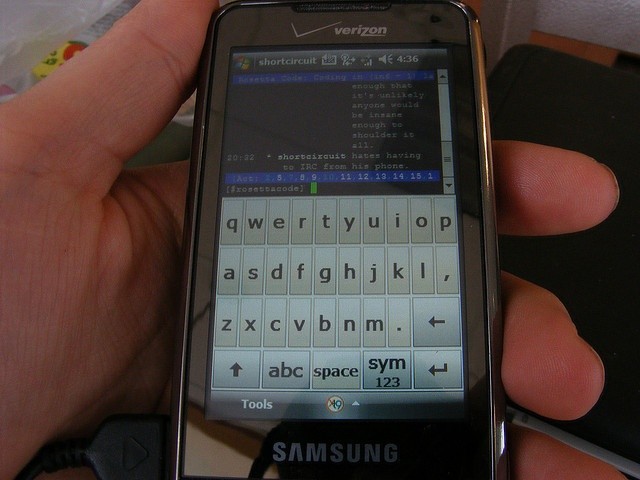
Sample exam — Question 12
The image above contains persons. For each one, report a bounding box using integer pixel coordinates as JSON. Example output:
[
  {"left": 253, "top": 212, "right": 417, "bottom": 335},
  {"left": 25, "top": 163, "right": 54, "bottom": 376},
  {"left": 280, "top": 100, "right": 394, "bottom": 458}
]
[{"left": 0, "top": 0, "right": 631, "bottom": 479}]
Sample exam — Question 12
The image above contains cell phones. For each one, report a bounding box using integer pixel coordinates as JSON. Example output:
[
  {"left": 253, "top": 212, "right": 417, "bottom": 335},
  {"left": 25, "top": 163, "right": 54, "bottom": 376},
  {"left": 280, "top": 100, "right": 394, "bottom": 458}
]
[{"left": 165, "top": 1, "right": 511, "bottom": 477}]
